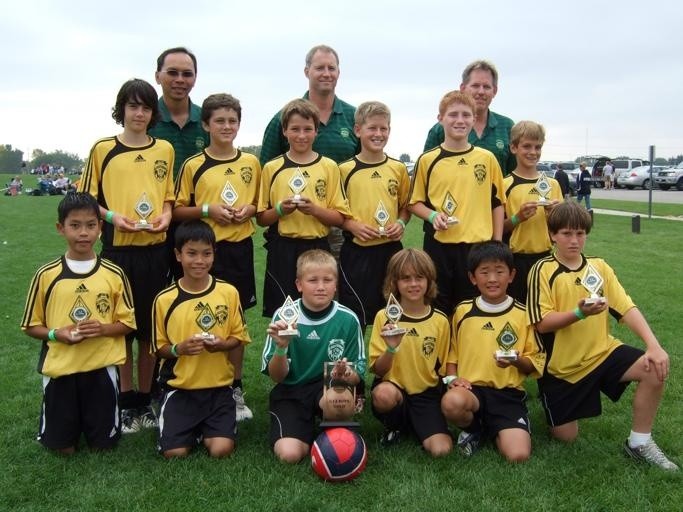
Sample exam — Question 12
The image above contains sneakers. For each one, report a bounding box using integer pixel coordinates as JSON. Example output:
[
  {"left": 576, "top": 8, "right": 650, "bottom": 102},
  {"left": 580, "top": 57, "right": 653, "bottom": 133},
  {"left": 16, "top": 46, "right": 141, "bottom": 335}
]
[
  {"left": 354, "top": 395, "right": 366, "bottom": 414},
  {"left": 380, "top": 423, "right": 402, "bottom": 447},
  {"left": 447, "top": 423, "right": 481, "bottom": 457},
  {"left": 623, "top": 436, "right": 680, "bottom": 471},
  {"left": 119, "top": 406, "right": 159, "bottom": 435},
  {"left": 232, "top": 387, "right": 253, "bottom": 422}
]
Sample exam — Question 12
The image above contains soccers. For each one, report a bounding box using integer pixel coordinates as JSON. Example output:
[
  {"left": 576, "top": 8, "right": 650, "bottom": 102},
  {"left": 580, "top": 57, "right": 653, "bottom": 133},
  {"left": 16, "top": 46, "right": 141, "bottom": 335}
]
[{"left": 310, "top": 428, "right": 366, "bottom": 483}]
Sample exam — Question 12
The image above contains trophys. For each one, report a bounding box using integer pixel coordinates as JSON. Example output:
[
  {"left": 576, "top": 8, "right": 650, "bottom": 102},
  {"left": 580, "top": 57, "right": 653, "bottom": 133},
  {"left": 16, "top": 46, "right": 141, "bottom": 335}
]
[
  {"left": 67, "top": 295, "right": 92, "bottom": 337},
  {"left": 580, "top": 263, "right": 606, "bottom": 306}
]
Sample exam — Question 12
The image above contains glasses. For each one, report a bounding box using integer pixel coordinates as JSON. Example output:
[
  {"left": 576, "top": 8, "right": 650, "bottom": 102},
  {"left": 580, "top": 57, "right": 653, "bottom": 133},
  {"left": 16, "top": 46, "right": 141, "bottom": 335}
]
[{"left": 161, "top": 70, "right": 193, "bottom": 77}]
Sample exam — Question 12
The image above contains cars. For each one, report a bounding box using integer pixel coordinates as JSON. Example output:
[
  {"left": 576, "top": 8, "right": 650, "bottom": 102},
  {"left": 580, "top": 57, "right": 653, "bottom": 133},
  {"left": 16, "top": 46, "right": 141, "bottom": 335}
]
[{"left": 537, "top": 155, "right": 683, "bottom": 197}]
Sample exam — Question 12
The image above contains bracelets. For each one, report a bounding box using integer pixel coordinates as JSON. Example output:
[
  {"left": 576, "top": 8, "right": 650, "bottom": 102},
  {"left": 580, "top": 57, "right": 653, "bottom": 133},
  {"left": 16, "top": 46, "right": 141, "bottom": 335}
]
[
  {"left": 105, "top": 211, "right": 114, "bottom": 223},
  {"left": 48, "top": 328, "right": 58, "bottom": 343},
  {"left": 429, "top": 212, "right": 438, "bottom": 223},
  {"left": 397, "top": 219, "right": 405, "bottom": 228},
  {"left": 511, "top": 215, "right": 518, "bottom": 225},
  {"left": 276, "top": 202, "right": 283, "bottom": 217},
  {"left": 574, "top": 308, "right": 586, "bottom": 320},
  {"left": 440, "top": 240, "right": 548, "bottom": 462},
  {"left": 202, "top": 204, "right": 208, "bottom": 217}
]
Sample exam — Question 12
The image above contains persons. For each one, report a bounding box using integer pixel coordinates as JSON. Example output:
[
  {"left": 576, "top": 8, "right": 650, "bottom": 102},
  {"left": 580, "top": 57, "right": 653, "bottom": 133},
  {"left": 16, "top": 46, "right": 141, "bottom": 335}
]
[
  {"left": 149, "top": 221, "right": 252, "bottom": 458},
  {"left": 338, "top": 100, "right": 412, "bottom": 330},
  {"left": 526, "top": 203, "right": 679, "bottom": 473},
  {"left": 259, "top": 45, "right": 363, "bottom": 172},
  {"left": 5, "top": 162, "right": 86, "bottom": 196},
  {"left": 407, "top": 91, "right": 508, "bottom": 318},
  {"left": 368, "top": 249, "right": 453, "bottom": 459},
  {"left": 500, "top": 120, "right": 562, "bottom": 305},
  {"left": 255, "top": 100, "right": 352, "bottom": 317},
  {"left": 144, "top": 47, "right": 209, "bottom": 397},
  {"left": 20, "top": 192, "right": 137, "bottom": 456},
  {"left": 171, "top": 93, "right": 263, "bottom": 424},
  {"left": 555, "top": 161, "right": 616, "bottom": 210},
  {"left": 78, "top": 78, "right": 177, "bottom": 434},
  {"left": 422, "top": 62, "right": 517, "bottom": 177},
  {"left": 261, "top": 249, "right": 368, "bottom": 463}
]
